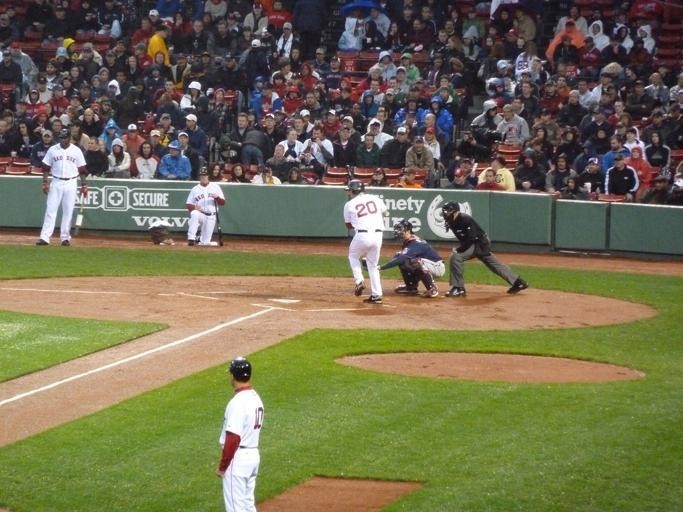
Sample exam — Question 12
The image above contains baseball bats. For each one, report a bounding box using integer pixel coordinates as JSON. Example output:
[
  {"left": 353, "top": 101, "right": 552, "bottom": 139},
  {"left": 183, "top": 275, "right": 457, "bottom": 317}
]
[
  {"left": 214, "top": 199, "right": 222, "bottom": 247},
  {"left": 75, "top": 192, "right": 84, "bottom": 238}
]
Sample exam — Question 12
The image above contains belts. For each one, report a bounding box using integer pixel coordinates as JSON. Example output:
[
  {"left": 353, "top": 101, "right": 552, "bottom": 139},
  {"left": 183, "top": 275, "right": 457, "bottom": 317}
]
[
  {"left": 358, "top": 230, "right": 381, "bottom": 232},
  {"left": 205, "top": 213, "right": 215, "bottom": 215},
  {"left": 52, "top": 176, "right": 78, "bottom": 180}
]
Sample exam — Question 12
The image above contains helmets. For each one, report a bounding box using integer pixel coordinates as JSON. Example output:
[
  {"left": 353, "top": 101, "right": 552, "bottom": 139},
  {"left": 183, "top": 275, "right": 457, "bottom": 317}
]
[
  {"left": 442, "top": 200, "right": 459, "bottom": 222},
  {"left": 348, "top": 179, "right": 364, "bottom": 191},
  {"left": 230, "top": 358, "right": 251, "bottom": 379},
  {"left": 200, "top": 167, "right": 209, "bottom": 176},
  {"left": 393, "top": 221, "right": 414, "bottom": 240},
  {"left": 58, "top": 129, "right": 69, "bottom": 139}
]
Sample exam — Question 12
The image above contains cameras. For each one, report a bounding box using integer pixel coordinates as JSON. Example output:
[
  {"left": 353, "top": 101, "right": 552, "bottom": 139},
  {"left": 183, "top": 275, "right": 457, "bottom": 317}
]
[{"left": 311, "top": 138, "right": 316, "bottom": 142}]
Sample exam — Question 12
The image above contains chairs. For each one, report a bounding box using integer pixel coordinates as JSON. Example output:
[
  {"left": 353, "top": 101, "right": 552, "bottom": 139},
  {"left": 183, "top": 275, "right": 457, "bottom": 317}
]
[{"left": 0, "top": 1, "right": 682, "bottom": 208}]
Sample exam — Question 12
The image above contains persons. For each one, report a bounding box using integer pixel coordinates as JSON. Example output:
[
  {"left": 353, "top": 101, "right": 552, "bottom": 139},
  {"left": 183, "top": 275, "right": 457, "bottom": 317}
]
[
  {"left": 0, "top": 0, "right": 682, "bottom": 206},
  {"left": 35, "top": 128, "right": 88, "bottom": 246},
  {"left": 377, "top": 219, "right": 445, "bottom": 297},
  {"left": 185, "top": 165, "right": 225, "bottom": 245},
  {"left": 441, "top": 201, "right": 527, "bottom": 296},
  {"left": 215, "top": 357, "right": 264, "bottom": 512},
  {"left": 342, "top": 179, "right": 385, "bottom": 304}
]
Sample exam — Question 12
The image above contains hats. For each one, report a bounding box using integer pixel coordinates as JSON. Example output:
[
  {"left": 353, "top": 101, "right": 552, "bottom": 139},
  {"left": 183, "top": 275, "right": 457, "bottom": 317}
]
[
  {"left": 414, "top": 136, "right": 423, "bottom": 143},
  {"left": 252, "top": 39, "right": 261, "bottom": 47},
  {"left": 316, "top": 48, "right": 325, "bottom": 55},
  {"left": 38, "top": 77, "right": 46, "bottom": 84},
  {"left": 133, "top": 44, "right": 145, "bottom": 50},
  {"left": 3, "top": 51, "right": 11, "bottom": 55},
  {"left": 426, "top": 128, "right": 434, "bottom": 133},
  {"left": 42, "top": 129, "right": 52, "bottom": 137},
  {"left": 341, "top": 116, "right": 353, "bottom": 122},
  {"left": 330, "top": 56, "right": 340, "bottom": 62},
  {"left": 168, "top": 114, "right": 197, "bottom": 149},
  {"left": 397, "top": 66, "right": 406, "bottom": 73},
  {"left": 266, "top": 113, "right": 274, "bottom": 119},
  {"left": 300, "top": 110, "right": 309, "bottom": 116},
  {"left": 128, "top": 123, "right": 137, "bottom": 132},
  {"left": 386, "top": 88, "right": 395, "bottom": 95},
  {"left": 397, "top": 127, "right": 406, "bottom": 135},
  {"left": 151, "top": 129, "right": 160, "bottom": 137},
  {"left": 283, "top": 22, "right": 292, "bottom": 30}
]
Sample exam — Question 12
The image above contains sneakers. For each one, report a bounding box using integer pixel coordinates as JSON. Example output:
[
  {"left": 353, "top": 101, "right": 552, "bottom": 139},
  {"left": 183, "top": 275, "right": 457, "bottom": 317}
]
[
  {"left": 508, "top": 279, "right": 527, "bottom": 293},
  {"left": 364, "top": 297, "right": 382, "bottom": 304},
  {"left": 36, "top": 239, "right": 47, "bottom": 245},
  {"left": 446, "top": 287, "right": 465, "bottom": 296},
  {"left": 423, "top": 287, "right": 438, "bottom": 297},
  {"left": 355, "top": 282, "right": 365, "bottom": 296},
  {"left": 396, "top": 287, "right": 419, "bottom": 293},
  {"left": 60, "top": 240, "right": 68, "bottom": 245}
]
